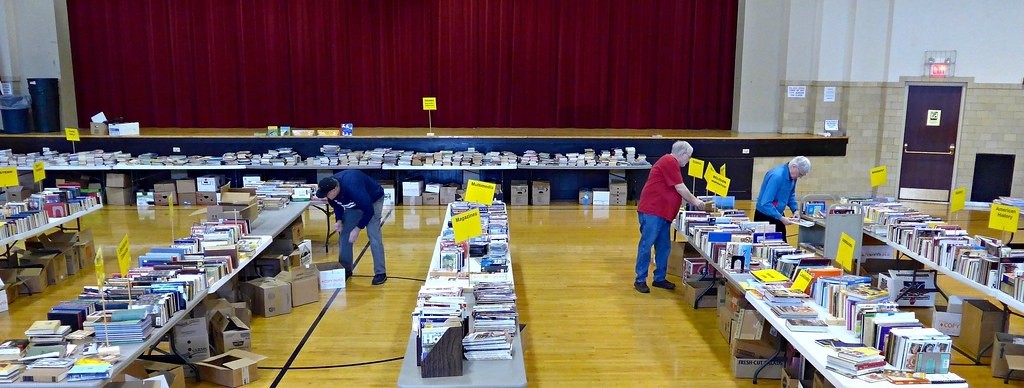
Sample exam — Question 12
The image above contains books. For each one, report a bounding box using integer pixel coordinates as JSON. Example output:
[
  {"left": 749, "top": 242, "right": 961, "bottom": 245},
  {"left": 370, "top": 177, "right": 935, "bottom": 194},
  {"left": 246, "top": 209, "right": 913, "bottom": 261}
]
[
  {"left": 0, "top": 147, "right": 305, "bottom": 167},
  {"left": 241, "top": 179, "right": 294, "bottom": 214},
  {"left": 675, "top": 196, "right": 1024, "bottom": 386},
  {"left": 0, "top": 219, "right": 262, "bottom": 383},
  {"left": 412, "top": 202, "right": 519, "bottom": 362},
  {"left": 300, "top": 145, "right": 647, "bottom": 167},
  {"left": 0, "top": 183, "right": 103, "bottom": 240}
]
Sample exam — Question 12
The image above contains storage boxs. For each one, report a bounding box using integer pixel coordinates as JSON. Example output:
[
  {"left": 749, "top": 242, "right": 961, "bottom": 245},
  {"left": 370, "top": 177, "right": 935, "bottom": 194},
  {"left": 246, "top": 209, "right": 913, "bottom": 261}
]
[
  {"left": 90, "top": 122, "right": 108, "bottom": 135},
  {"left": 108, "top": 122, "right": 139, "bottom": 135},
  {"left": 0, "top": 174, "right": 1024, "bottom": 388}
]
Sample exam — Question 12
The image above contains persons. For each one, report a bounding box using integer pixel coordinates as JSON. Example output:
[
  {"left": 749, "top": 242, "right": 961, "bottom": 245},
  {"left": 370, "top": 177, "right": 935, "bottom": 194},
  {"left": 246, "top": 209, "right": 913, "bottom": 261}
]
[
  {"left": 633, "top": 141, "right": 705, "bottom": 293},
  {"left": 316, "top": 170, "right": 387, "bottom": 286},
  {"left": 754, "top": 156, "right": 811, "bottom": 244}
]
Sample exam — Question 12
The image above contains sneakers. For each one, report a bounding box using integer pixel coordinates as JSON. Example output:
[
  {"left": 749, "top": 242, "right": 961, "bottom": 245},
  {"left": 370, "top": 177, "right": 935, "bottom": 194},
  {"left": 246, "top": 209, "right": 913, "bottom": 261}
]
[{"left": 372, "top": 273, "right": 387, "bottom": 285}]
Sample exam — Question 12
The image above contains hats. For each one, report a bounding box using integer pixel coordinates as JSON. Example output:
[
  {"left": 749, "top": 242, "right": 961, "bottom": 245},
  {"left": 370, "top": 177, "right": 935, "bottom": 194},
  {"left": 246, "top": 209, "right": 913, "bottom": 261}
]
[{"left": 316, "top": 177, "right": 337, "bottom": 198}]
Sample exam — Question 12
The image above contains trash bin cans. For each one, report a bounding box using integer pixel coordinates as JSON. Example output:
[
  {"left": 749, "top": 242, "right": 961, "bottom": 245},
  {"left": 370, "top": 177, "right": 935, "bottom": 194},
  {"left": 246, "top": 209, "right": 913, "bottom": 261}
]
[
  {"left": 0, "top": 94, "right": 32, "bottom": 134},
  {"left": 26, "top": 78, "right": 61, "bottom": 133}
]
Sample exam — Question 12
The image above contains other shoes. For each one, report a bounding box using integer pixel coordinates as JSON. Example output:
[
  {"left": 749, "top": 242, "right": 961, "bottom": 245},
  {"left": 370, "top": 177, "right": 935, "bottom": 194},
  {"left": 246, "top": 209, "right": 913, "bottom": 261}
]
[
  {"left": 634, "top": 282, "right": 650, "bottom": 293},
  {"left": 652, "top": 279, "right": 675, "bottom": 289}
]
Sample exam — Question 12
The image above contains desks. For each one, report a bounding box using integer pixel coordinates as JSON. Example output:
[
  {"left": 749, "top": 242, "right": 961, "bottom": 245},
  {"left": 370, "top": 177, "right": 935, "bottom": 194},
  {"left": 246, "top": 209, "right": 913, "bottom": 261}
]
[
  {"left": 0, "top": 203, "right": 103, "bottom": 296},
  {"left": 0, "top": 199, "right": 315, "bottom": 388},
  {"left": 669, "top": 219, "right": 968, "bottom": 388},
  {"left": 398, "top": 205, "right": 526, "bottom": 388},
  {"left": 800, "top": 196, "right": 1024, "bottom": 384}
]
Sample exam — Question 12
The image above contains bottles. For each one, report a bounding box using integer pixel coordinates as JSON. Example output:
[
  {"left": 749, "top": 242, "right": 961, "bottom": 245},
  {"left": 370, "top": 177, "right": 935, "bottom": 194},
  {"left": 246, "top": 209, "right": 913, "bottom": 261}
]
[
  {"left": 184, "top": 201, "right": 191, "bottom": 206},
  {"left": 789, "top": 352, "right": 799, "bottom": 379},
  {"left": 582, "top": 187, "right": 589, "bottom": 204},
  {"left": 282, "top": 197, "right": 286, "bottom": 207}
]
